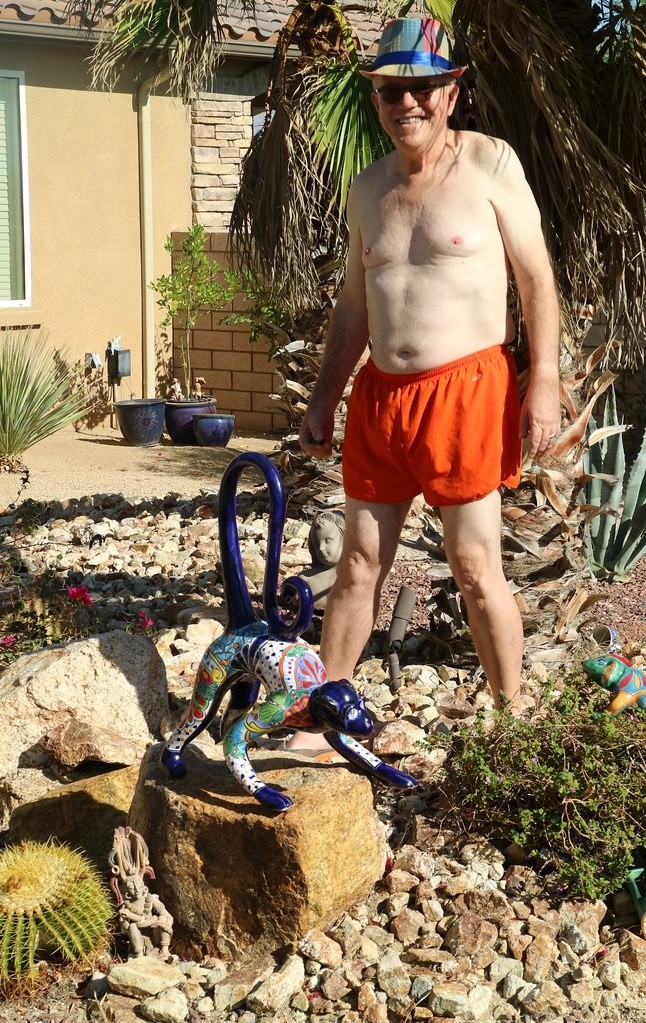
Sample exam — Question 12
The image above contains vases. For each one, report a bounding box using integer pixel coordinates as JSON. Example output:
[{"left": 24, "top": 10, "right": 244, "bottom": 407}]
[
  {"left": 113, "top": 399, "right": 167, "bottom": 447},
  {"left": 192, "top": 413, "right": 235, "bottom": 447}
]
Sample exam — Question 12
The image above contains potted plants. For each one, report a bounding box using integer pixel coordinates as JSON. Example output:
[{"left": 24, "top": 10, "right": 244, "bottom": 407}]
[{"left": 147, "top": 221, "right": 243, "bottom": 449}]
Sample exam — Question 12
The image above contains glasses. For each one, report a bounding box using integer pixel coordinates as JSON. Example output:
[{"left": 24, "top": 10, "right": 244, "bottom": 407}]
[{"left": 374, "top": 79, "right": 456, "bottom": 105}]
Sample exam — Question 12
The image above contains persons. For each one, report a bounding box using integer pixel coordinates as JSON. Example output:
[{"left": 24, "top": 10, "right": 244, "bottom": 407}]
[{"left": 299, "top": 20, "right": 561, "bottom": 717}]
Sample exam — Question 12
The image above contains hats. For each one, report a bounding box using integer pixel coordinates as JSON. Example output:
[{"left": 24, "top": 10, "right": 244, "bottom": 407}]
[{"left": 358, "top": 17, "right": 467, "bottom": 79}]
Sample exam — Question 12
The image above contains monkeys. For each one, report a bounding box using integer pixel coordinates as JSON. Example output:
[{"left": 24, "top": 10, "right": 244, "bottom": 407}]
[{"left": 157, "top": 450, "right": 418, "bottom": 813}]
